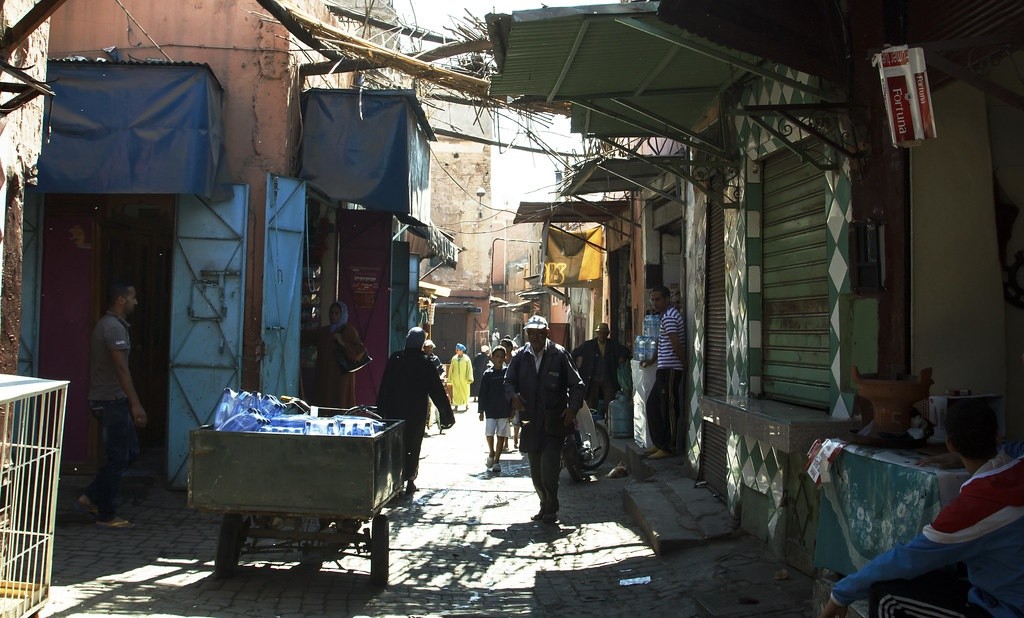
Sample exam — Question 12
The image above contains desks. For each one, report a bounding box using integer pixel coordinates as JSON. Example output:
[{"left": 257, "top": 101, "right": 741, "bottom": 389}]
[{"left": 813, "top": 441, "right": 976, "bottom": 578}]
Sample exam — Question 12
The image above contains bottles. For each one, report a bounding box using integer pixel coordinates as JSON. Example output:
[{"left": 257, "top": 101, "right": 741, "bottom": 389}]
[
  {"left": 633, "top": 314, "right": 661, "bottom": 362},
  {"left": 214, "top": 388, "right": 388, "bottom": 438}
]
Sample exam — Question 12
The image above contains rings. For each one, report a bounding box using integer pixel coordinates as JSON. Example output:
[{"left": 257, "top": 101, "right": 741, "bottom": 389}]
[{"left": 137, "top": 418, "right": 141, "bottom": 423}]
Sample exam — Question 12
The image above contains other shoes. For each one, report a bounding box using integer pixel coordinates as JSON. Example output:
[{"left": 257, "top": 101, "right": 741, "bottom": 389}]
[
  {"left": 642, "top": 445, "right": 676, "bottom": 459},
  {"left": 492, "top": 463, "right": 501, "bottom": 471},
  {"left": 486, "top": 452, "right": 495, "bottom": 468},
  {"left": 531, "top": 512, "right": 556, "bottom": 522},
  {"left": 406, "top": 485, "right": 419, "bottom": 493}
]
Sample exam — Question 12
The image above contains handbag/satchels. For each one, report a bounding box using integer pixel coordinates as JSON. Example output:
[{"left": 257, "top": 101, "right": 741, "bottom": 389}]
[{"left": 337, "top": 326, "right": 373, "bottom": 374}]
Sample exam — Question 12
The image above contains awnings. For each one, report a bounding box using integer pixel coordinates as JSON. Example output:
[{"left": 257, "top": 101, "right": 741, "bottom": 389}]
[
  {"left": 490, "top": 274, "right": 570, "bottom": 313},
  {"left": 485, "top": 0, "right": 904, "bottom": 223},
  {"left": 542, "top": 225, "right": 602, "bottom": 288},
  {"left": 419, "top": 281, "right": 451, "bottom": 298},
  {"left": 400, "top": 217, "right": 454, "bottom": 272}
]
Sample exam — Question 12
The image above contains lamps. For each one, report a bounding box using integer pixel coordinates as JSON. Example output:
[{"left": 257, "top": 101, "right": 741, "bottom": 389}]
[{"left": 476, "top": 187, "right": 486, "bottom": 218}]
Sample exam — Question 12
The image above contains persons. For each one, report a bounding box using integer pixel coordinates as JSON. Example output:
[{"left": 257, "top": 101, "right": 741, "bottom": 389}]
[
  {"left": 79, "top": 284, "right": 146, "bottom": 528},
  {"left": 478, "top": 345, "right": 515, "bottom": 472},
  {"left": 491, "top": 328, "right": 500, "bottom": 351},
  {"left": 372, "top": 327, "right": 455, "bottom": 492},
  {"left": 821, "top": 401, "right": 1024, "bottom": 618},
  {"left": 472, "top": 346, "right": 492, "bottom": 402},
  {"left": 422, "top": 340, "right": 444, "bottom": 437},
  {"left": 448, "top": 343, "right": 474, "bottom": 411},
  {"left": 300, "top": 301, "right": 365, "bottom": 417},
  {"left": 499, "top": 334, "right": 519, "bottom": 452},
  {"left": 503, "top": 315, "right": 586, "bottom": 520},
  {"left": 571, "top": 323, "right": 632, "bottom": 422},
  {"left": 643, "top": 285, "right": 687, "bottom": 458}
]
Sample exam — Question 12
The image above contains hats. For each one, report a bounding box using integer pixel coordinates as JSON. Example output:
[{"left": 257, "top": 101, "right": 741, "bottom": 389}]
[
  {"left": 456, "top": 343, "right": 467, "bottom": 352},
  {"left": 423, "top": 340, "right": 435, "bottom": 348},
  {"left": 523, "top": 315, "right": 549, "bottom": 330},
  {"left": 595, "top": 323, "right": 611, "bottom": 332}
]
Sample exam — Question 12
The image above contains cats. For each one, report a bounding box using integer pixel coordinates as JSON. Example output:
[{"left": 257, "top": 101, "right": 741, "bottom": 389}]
[{"left": 606, "top": 461, "right": 630, "bottom": 478}]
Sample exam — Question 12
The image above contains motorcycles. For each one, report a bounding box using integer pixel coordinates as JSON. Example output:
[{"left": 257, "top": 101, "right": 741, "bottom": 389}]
[
  {"left": 186, "top": 395, "right": 406, "bottom": 588},
  {"left": 559, "top": 405, "right": 612, "bottom": 485}
]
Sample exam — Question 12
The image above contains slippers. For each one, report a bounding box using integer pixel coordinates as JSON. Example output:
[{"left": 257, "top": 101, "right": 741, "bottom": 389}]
[
  {"left": 95, "top": 518, "right": 136, "bottom": 529},
  {"left": 75, "top": 496, "right": 99, "bottom": 518}
]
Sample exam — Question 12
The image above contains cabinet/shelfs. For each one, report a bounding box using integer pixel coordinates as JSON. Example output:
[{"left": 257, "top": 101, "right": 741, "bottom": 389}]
[{"left": 299, "top": 265, "right": 321, "bottom": 368}]
